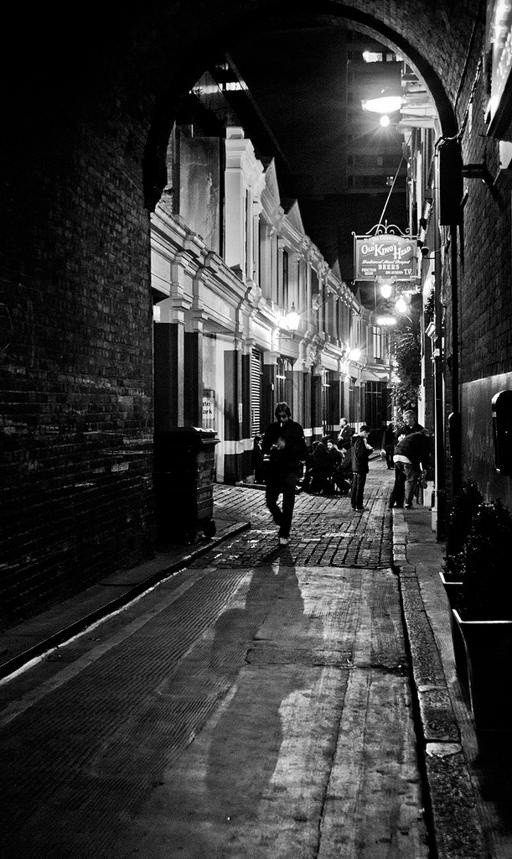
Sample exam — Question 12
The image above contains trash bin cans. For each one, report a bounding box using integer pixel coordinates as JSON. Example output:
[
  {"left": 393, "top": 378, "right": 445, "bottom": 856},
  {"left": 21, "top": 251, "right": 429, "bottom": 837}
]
[{"left": 156, "top": 426, "right": 220, "bottom": 546}]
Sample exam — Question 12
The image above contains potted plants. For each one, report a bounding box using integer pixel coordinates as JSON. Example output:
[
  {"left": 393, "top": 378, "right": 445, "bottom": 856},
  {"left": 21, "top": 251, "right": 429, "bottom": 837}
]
[
  {"left": 450, "top": 500, "right": 512, "bottom": 762},
  {"left": 439, "top": 481, "right": 483, "bottom": 679}
]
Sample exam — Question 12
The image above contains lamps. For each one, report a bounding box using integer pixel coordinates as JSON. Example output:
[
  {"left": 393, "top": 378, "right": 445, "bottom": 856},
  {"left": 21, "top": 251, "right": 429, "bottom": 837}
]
[
  {"left": 274, "top": 301, "right": 301, "bottom": 340},
  {"left": 360, "top": 87, "right": 438, "bottom": 121}
]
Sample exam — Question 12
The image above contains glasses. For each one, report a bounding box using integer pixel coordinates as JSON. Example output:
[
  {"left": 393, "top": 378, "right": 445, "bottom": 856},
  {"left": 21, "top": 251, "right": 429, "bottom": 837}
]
[{"left": 276, "top": 414, "right": 288, "bottom": 419}]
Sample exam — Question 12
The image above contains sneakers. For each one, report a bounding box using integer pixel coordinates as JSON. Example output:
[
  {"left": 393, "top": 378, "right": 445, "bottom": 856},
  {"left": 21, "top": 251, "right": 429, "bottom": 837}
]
[
  {"left": 278, "top": 537, "right": 292, "bottom": 544},
  {"left": 405, "top": 504, "right": 415, "bottom": 509},
  {"left": 353, "top": 507, "right": 369, "bottom": 513}
]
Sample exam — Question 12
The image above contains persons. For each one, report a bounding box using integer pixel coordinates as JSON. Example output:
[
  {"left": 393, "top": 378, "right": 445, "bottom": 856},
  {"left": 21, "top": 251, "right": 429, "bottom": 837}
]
[
  {"left": 263, "top": 401, "right": 307, "bottom": 549},
  {"left": 251, "top": 410, "right": 435, "bottom": 513}
]
